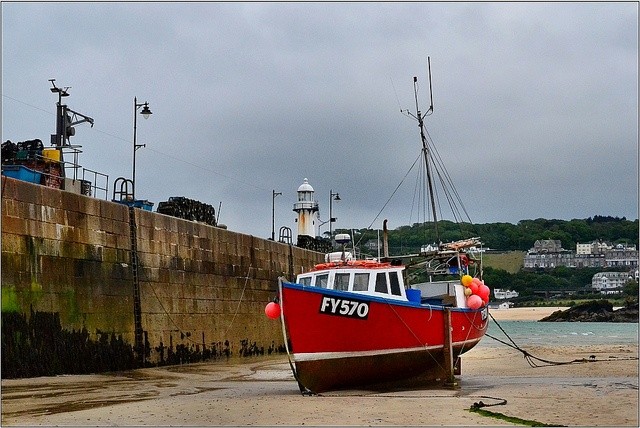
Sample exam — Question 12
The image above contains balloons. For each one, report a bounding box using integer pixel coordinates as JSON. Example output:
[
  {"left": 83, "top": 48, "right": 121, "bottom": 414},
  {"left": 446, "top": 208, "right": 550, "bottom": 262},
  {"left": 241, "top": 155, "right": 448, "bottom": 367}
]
[
  {"left": 473, "top": 278, "right": 483, "bottom": 287},
  {"left": 469, "top": 282, "right": 478, "bottom": 293},
  {"left": 264, "top": 302, "right": 281, "bottom": 320},
  {"left": 484, "top": 296, "right": 489, "bottom": 304},
  {"left": 461, "top": 275, "right": 472, "bottom": 286},
  {"left": 477, "top": 285, "right": 490, "bottom": 298},
  {"left": 481, "top": 301, "right": 485, "bottom": 308},
  {"left": 467, "top": 294, "right": 482, "bottom": 310}
]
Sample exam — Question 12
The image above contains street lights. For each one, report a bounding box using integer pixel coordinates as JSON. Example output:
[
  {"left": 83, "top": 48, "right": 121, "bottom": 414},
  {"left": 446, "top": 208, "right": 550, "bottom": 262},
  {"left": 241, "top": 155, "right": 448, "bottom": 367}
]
[
  {"left": 132, "top": 97, "right": 152, "bottom": 201},
  {"left": 330, "top": 189, "right": 341, "bottom": 240},
  {"left": 272, "top": 190, "right": 282, "bottom": 240}
]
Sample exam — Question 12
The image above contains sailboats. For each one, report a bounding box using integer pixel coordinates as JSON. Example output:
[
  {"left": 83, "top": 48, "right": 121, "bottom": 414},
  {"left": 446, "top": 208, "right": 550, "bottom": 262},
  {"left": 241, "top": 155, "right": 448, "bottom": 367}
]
[{"left": 278, "top": 57, "right": 489, "bottom": 393}]
